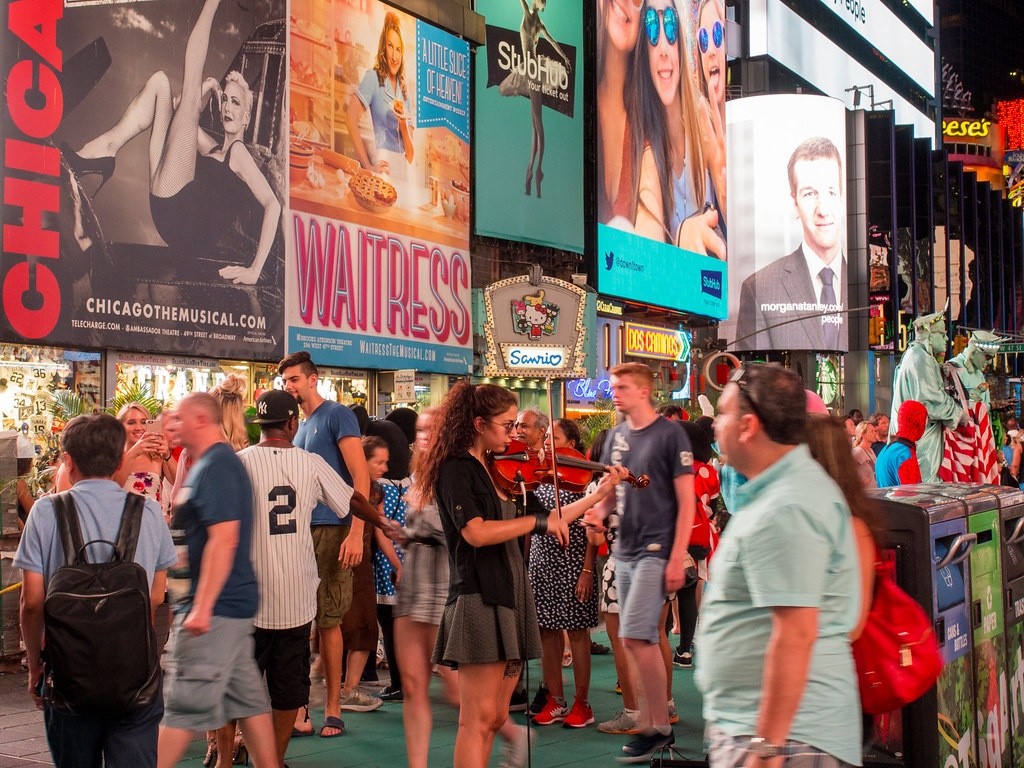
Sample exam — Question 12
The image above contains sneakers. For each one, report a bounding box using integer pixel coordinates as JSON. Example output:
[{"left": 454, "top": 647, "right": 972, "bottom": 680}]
[
  {"left": 508, "top": 689, "right": 528, "bottom": 711},
  {"left": 597, "top": 709, "right": 643, "bottom": 731},
  {"left": 524, "top": 682, "right": 550, "bottom": 716},
  {"left": 668, "top": 699, "right": 680, "bottom": 724},
  {"left": 562, "top": 698, "right": 594, "bottom": 729},
  {"left": 339, "top": 689, "right": 383, "bottom": 712},
  {"left": 531, "top": 698, "right": 569, "bottom": 726},
  {"left": 369, "top": 686, "right": 404, "bottom": 703}
]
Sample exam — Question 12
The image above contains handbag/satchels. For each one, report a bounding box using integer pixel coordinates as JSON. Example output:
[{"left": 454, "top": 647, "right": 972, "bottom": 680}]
[
  {"left": 847, "top": 520, "right": 944, "bottom": 753},
  {"left": 681, "top": 551, "right": 699, "bottom": 587}
]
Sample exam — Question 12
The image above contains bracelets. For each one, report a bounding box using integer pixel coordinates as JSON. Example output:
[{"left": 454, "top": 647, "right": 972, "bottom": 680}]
[
  {"left": 583, "top": 569, "right": 593, "bottom": 574},
  {"left": 164, "top": 453, "right": 171, "bottom": 461},
  {"left": 531, "top": 511, "right": 549, "bottom": 532}
]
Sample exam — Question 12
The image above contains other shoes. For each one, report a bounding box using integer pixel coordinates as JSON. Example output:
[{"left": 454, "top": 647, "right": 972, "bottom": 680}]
[
  {"left": 622, "top": 724, "right": 676, "bottom": 755},
  {"left": 498, "top": 725, "right": 535, "bottom": 768},
  {"left": 560, "top": 641, "right": 691, "bottom": 695}
]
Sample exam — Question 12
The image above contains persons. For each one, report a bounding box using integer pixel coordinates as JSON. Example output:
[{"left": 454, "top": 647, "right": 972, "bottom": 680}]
[
  {"left": 597, "top": 0, "right": 726, "bottom": 261},
  {"left": 278, "top": 351, "right": 372, "bottom": 736},
  {"left": 59, "top": 0, "right": 281, "bottom": 286},
  {"left": 345, "top": 10, "right": 414, "bottom": 177},
  {"left": 874, "top": 400, "right": 929, "bottom": 488},
  {"left": 498, "top": 0, "right": 570, "bottom": 198},
  {"left": 11, "top": 314, "right": 1024, "bottom": 768},
  {"left": 736, "top": 136, "right": 848, "bottom": 352}
]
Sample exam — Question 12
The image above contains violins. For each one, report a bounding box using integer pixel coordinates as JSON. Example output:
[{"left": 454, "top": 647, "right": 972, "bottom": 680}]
[{"left": 486, "top": 438, "right": 651, "bottom": 493}]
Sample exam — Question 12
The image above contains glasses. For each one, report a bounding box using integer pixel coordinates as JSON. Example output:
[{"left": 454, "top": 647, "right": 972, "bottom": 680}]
[
  {"left": 698, "top": 20, "right": 724, "bottom": 56},
  {"left": 730, "top": 370, "right": 765, "bottom": 426},
  {"left": 486, "top": 419, "right": 515, "bottom": 434},
  {"left": 643, "top": 5, "right": 679, "bottom": 47}
]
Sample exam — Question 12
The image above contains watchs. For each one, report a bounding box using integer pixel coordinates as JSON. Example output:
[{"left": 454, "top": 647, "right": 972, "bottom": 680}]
[{"left": 746, "top": 737, "right": 791, "bottom": 758}]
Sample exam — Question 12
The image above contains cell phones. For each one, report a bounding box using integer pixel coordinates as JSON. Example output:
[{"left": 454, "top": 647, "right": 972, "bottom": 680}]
[{"left": 146, "top": 420, "right": 163, "bottom": 441}]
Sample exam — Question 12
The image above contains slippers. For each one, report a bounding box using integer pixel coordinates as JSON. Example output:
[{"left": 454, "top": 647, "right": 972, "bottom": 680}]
[
  {"left": 320, "top": 716, "right": 345, "bottom": 738},
  {"left": 291, "top": 726, "right": 315, "bottom": 738}
]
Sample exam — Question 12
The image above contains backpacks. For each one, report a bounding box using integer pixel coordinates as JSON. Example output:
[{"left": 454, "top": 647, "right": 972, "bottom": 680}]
[
  {"left": 688, "top": 473, "right": 710, "bottom": 559},
  {"left": 38, "top": 489, "right": 166, "bottom": 726}
]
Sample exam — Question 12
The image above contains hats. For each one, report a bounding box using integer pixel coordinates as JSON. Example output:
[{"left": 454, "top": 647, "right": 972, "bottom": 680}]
[{"left": 246, "top": 388, "right": 299, "bottom": 424}]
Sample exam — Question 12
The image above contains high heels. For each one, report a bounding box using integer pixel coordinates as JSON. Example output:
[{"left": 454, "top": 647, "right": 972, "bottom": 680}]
[
  {"left": 57, "top": 140, "right": 117, "bottom": 202},
  {"left": 203, "top": 730, "right": 249, "bottom": 768}
]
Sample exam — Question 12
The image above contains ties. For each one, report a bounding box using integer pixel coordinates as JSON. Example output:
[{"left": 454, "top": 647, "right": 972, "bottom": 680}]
[{"left": 819, "top": 268, "right": 840, "bottom": 351}]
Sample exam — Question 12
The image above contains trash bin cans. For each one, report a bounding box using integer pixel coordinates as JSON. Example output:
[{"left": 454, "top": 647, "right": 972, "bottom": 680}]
[{"left": 862, "top": 481, "right": 1024, "bottom": 768}]
